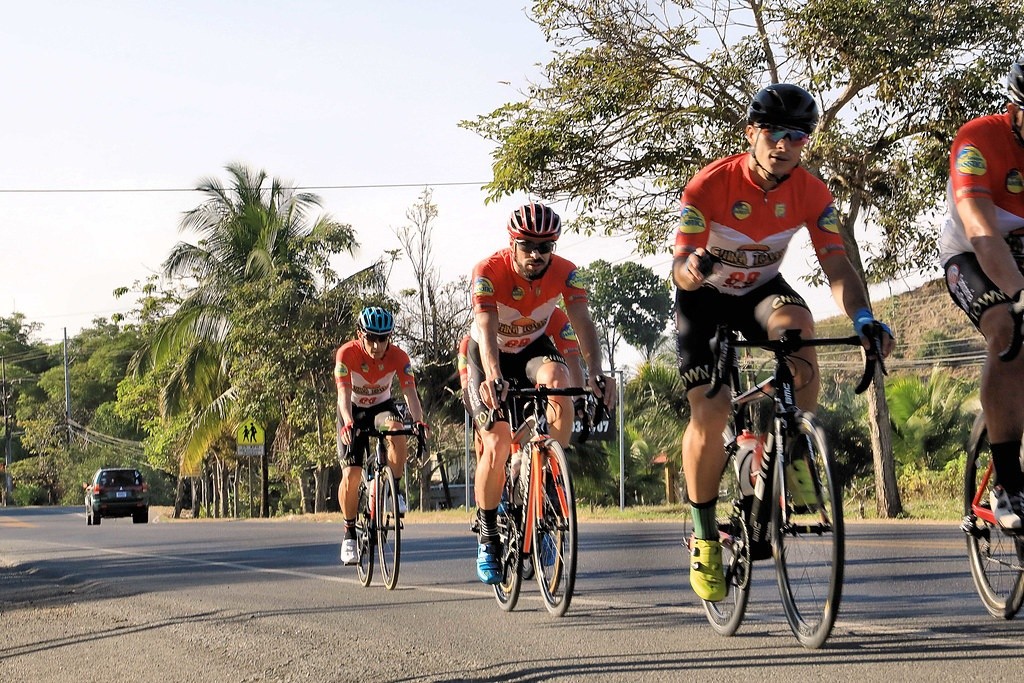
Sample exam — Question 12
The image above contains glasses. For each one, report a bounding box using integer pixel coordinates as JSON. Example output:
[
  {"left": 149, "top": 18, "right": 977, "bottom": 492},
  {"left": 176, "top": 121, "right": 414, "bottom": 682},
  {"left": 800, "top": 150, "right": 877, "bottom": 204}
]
[
  {"left": 751, "top": 121, "right": 809, "bottom": 147},
  {"left": 360, "top": 328, "right": 390, "bottom": 343},
  {"left": 512, "top": 237, "right": 555, "bottom": 255}
]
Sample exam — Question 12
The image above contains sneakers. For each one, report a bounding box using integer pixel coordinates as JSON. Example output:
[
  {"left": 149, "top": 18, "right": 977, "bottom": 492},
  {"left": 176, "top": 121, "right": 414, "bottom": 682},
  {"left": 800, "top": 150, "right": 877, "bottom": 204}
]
[
  {"left": 689, "top": 529, "right": 727, "bottom": 601},
  {"left": 987, "top": 478, "right": 1024, "bottom": 534},
  {"left": 541, "top": 531, "right": 557, "bottom": 567},
  {"left": 389, "top": 494, "right": 407, "bottom": 514},
  {"left": 476, "top": 535, "right": 505, "bottom": 584},
  {"left": 782, "top": 456, "right": 824, "bottom": 526},
  {"left": 340, "top": 536, "right": 359, "bottom": 564}
]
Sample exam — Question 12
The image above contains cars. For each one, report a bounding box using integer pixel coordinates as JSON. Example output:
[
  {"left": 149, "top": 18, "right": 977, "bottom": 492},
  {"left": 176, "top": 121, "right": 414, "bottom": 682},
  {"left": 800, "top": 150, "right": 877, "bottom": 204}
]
[{"left": 83, "top": 467, "right": 148, "bottom": 525}]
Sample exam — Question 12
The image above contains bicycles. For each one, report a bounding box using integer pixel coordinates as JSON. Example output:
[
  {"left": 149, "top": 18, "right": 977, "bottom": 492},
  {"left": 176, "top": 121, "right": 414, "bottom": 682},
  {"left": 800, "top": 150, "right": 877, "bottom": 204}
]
[
  {"left": 683, "top": 320, "right": 889, "bottom": 648},
  {"left": 471, "top": 374, "right": 612, "bottom": 619},
  {"left": 959, "top": 289, "right": 1024, "bottom": 619},
  {"left": 343, "top": 421, "right": 427, "bottom": 590}
]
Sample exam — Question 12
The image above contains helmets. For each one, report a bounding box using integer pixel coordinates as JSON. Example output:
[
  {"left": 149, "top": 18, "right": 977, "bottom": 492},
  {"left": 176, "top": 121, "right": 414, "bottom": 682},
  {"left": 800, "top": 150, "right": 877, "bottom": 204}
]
[
  {"left": 357, "top": 307, "right": 394, "bottom": 335},
  {"left": 1004, "top": 55, "right": 1024, "bottom": 108},
  {"left": 746, "top": 83, "right": 820, "bottom": 137},
  {"left": 507, "top": 202, "right": 561, "bottom": 242}
]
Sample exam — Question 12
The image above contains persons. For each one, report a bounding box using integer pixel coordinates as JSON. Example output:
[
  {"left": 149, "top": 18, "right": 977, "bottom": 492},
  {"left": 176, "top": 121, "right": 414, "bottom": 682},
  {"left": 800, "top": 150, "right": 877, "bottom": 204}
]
[
  {"left": 672, "top": 84, "right": 896, "bottom": 603},
  {"left": 334, "top": 306, "right": 433, "bottom": 563},
  {"left": 940, "top": 64, "right": 1024, "bottom": 537},
  {"left": 457, "top": 203, "right": 616, "bottom": 585}
]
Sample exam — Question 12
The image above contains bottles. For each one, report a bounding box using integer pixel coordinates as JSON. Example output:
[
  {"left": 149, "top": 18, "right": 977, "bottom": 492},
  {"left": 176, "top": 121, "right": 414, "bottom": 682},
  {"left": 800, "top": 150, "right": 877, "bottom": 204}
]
[
  {"left": 510, "top": 448, "right": 522, "bottom": 479},
  {"left": 750, "top": 433, "right": 765, "bottom": 485},
  {"left": 520, "top": 443, "right": 530, "bottom": 481},
  {"left": 369, "top": 478, "right": 374, "bottom": 510},
  {"left": 735, "top": 429, "right": 758, "bottom": 498}
]
